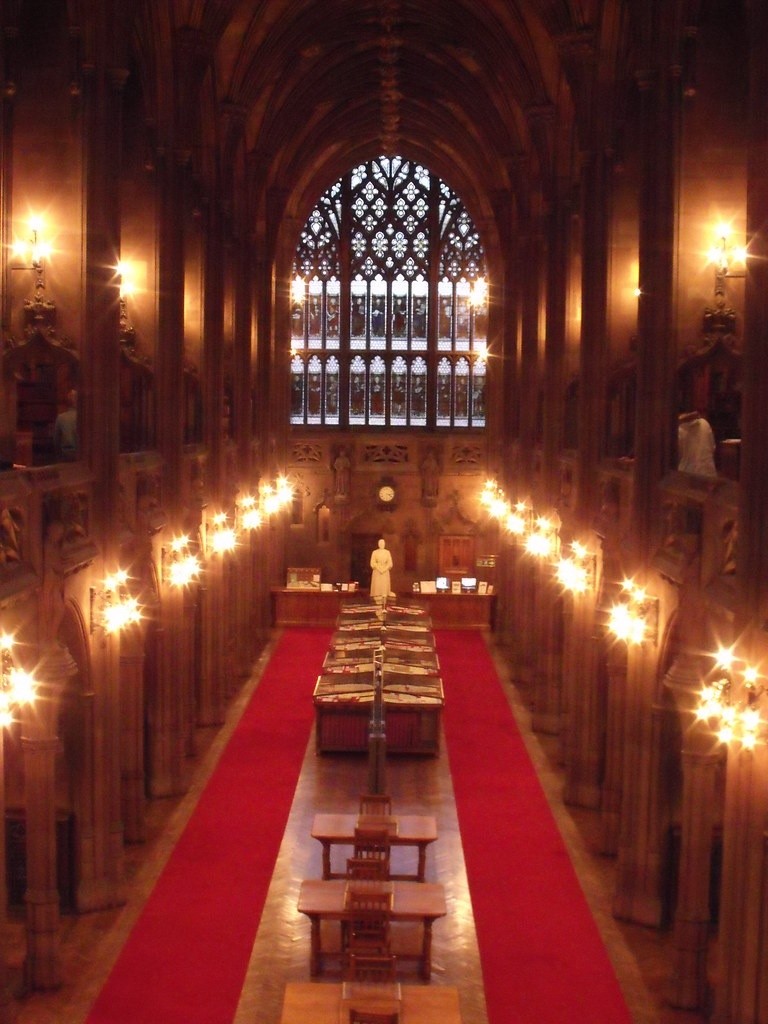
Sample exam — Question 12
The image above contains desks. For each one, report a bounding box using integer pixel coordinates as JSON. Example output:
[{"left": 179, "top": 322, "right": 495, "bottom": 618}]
[
  {"left": 271, "top": 585, "right": 359, "bottom": 625},
  {"left": 281, "top": 982, "right": 462, "bottom": 1024},
  {"left": 297, "top": 878, "right": 448, "bottom": 982},
  {"left": 311, "top": 814, "right": 438, "bottom": 882},
  {"left": 397, "top": 586, "right": 497, "bottom": 630}
]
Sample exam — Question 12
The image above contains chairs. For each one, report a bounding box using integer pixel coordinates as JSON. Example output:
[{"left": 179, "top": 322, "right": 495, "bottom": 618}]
[
  {"left": 360, "top": 795, "right": 392, "bottom": 814},
  {"left": 354, "top": 828, "right": 390, "bottom": 860},
  {"left": 350, "top": 1008, "right": 398, "bottom": 1024},
  {"left": 347, "top": 889, "right": 398, "bottom": 982},
  {"left": 341, "top": 858, "right": 389, "bottom": 952}
]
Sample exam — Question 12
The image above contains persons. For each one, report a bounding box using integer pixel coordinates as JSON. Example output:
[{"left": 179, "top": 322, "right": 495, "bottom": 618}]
[{"left": 369, "top": 538, "right": 394, "bottom": 600}]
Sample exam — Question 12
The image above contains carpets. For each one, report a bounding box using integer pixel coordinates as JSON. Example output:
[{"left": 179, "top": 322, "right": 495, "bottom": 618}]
[
  {"left": 82, "top": 625, "right": 338, "bottom": 1024},
  {"left": 433, "top": 630, "right": 636, "bottom": 1024}
]
